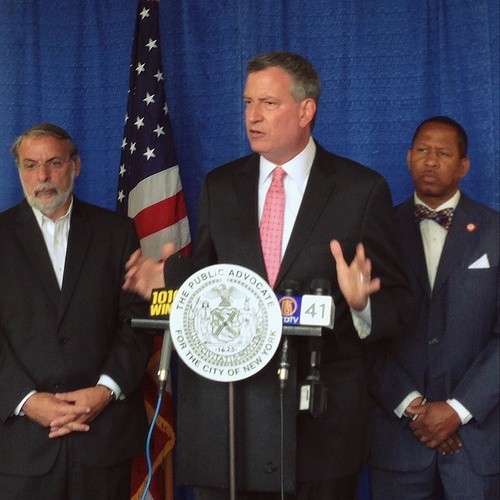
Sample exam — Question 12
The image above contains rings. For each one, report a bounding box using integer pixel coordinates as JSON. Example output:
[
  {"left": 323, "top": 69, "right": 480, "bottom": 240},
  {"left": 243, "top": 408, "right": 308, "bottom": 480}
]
[{"left": 359, "top": 272, "right": 364, "bottom": 283}]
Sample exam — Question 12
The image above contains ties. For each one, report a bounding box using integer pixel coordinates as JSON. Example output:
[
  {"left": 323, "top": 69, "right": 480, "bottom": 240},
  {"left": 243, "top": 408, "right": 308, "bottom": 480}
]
[{"left": 260, "top": 167, "right": 286, "bottom": 290}]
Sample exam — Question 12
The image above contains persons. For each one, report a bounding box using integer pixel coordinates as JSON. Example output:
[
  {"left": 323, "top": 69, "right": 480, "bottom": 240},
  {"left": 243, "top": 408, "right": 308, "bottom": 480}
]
[
  {"left": 0, "top": 122, "right": 156, "bottom": 499},
  {"left": 364, "top": 115, "right": 500, "bottom": 500},
  {"left": 123, "top": 52, "right": 429, "bottom": 500}
]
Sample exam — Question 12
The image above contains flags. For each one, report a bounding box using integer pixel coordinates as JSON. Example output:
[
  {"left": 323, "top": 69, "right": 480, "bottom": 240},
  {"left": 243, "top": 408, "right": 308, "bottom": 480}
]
[{"left": 116, "top": 0, "right": 192, "bottom": 263}]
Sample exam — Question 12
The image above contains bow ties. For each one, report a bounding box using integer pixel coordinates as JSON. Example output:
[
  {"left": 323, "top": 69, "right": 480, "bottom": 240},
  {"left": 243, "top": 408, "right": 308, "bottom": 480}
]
[{"left": 413, "top": 203, "right": 454, "bottom": 233}]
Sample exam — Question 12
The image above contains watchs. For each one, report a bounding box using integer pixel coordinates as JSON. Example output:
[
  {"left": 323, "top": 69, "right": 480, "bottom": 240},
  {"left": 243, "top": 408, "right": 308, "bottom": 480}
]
[{"left": 98, "top": 385, "right": 116, "bottom": 403}]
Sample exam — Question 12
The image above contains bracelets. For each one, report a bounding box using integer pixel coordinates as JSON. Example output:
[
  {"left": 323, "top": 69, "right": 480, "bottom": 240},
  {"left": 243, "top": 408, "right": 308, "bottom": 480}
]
[{"left": 413, "top": 397, "right": 427, "bottom": 421}]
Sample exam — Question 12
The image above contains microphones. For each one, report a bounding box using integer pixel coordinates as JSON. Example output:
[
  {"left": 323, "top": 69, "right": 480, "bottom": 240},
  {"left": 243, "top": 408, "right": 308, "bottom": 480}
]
[
  {"left": 276, "top": 280, "right": 302, "bottom": 393},
  {"left": 150, "top": 254, "right": 193, "bottom": 396},
  {"left": 299, "top": 279, "right": 336, "bottom": 419}
]
